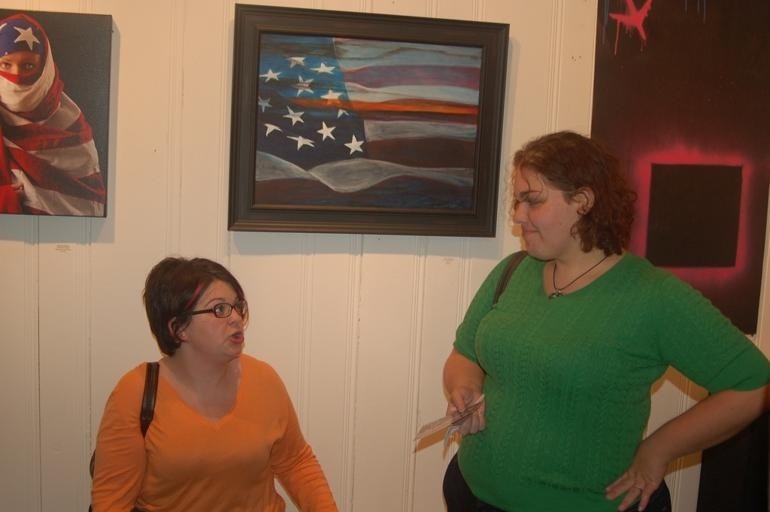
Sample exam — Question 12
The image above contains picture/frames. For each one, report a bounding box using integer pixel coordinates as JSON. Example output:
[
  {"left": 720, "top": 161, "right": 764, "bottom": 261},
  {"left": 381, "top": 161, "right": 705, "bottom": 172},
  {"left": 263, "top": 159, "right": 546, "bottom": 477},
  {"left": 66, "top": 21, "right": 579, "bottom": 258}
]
[{"left": 226, "top": 3, "right": 510, "bottom": 238}]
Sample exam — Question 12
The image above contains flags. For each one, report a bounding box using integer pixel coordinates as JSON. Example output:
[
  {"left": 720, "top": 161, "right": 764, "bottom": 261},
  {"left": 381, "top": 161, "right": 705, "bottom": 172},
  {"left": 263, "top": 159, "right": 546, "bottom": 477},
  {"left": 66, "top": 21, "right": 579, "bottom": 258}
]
[{"left": 253, "top": 32, "right": 482, "bottom": 208}]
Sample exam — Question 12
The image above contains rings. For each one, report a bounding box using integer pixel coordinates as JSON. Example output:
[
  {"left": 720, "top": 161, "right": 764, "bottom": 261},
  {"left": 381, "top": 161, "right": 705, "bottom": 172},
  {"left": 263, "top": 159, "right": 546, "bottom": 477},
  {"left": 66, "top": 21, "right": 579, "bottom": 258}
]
[{"left": 633, "top": 484, "right": 643, "bottom": 491}]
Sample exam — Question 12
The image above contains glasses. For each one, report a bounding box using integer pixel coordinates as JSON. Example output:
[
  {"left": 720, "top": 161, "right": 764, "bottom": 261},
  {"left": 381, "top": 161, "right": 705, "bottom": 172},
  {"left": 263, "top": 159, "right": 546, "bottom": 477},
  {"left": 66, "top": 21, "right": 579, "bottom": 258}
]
[{"left": 188, "top": 298, "right": 249, "bottom": 319}]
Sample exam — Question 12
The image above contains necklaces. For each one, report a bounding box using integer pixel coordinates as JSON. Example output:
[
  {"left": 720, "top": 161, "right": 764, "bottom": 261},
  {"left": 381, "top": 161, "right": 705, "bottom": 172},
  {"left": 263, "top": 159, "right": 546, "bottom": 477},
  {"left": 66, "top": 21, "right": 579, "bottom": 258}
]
[{"left": 549, "top": 255, "right": 607, "bottom": 300}]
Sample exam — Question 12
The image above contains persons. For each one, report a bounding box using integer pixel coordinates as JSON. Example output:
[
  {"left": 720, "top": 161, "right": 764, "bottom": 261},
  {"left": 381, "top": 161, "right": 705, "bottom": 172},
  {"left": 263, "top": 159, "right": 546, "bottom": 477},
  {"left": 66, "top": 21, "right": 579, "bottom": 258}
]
[
  {"left": 0, "top": 15, "right": 106, "bottom": 216},
  {"left": 90, "top": 257, "right": 339, "bottom": 512},
  {"left": 441, "top": 131, "right": 770, "bottom": 512}
]
